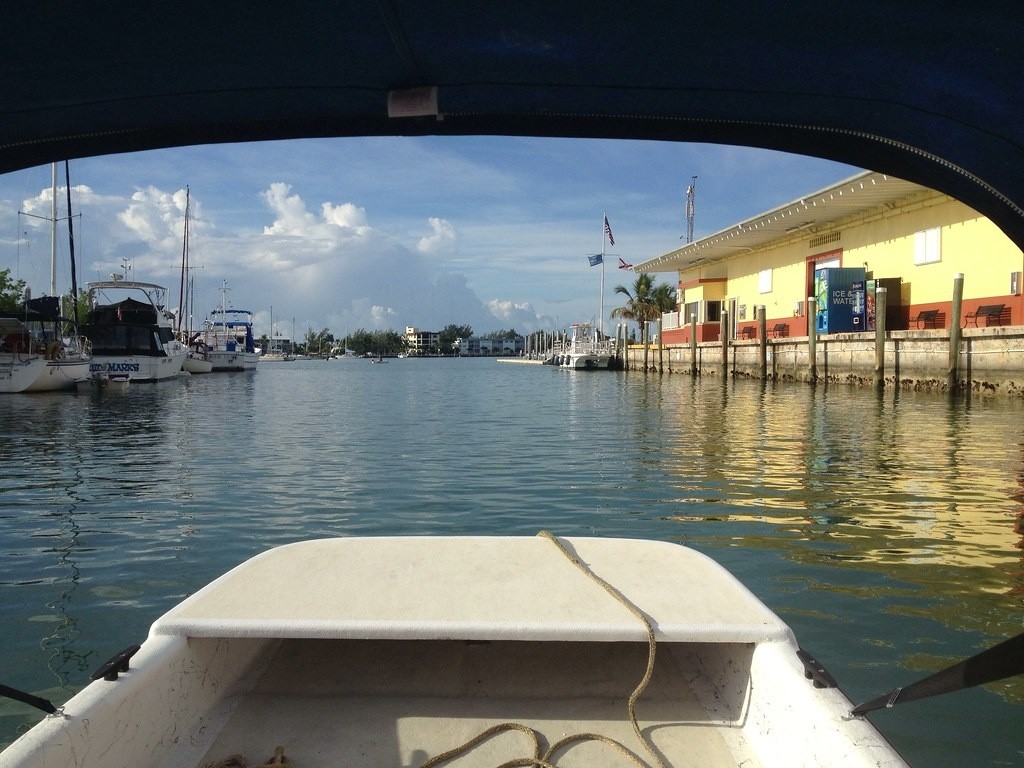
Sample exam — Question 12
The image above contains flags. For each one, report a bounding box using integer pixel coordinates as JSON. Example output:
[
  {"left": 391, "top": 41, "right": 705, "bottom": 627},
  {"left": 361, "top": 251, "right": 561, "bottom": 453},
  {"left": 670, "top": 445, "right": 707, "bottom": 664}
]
[
  {"left": 618, "top": 257, "right": 633, "bottom": 270},
  {"left": 588, "top": 254, "right": 603, "bottom": 266},
  {"left": 604, "top": 216, "right": 615, "bottom": 247}
]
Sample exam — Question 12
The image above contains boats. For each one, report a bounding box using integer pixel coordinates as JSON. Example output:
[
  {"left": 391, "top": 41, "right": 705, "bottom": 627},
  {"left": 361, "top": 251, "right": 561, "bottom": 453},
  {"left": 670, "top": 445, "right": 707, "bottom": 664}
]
[
  {"left": 283, "top": 356, "right": 297, "bottom": 362},
  {"left": 328, "top": 355, "right": 338, "bottom": 360},
  {"left": 200, "top": 280, "right": 263, "bottom": 372},
  {"left": 551, "top": 323, "right": 615, "bottom": 372},
  {"left": 78, "top": 256, "right": 191, "bottom": 385}
]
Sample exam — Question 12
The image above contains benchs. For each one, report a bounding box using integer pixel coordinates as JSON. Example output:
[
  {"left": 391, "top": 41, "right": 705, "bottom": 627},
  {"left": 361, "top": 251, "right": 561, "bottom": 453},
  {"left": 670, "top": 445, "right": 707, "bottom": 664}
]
[
  {"left": 766, "top": 322, "right": 786, "bottom": 337},
  {"left": 964, "top": 303, "right": 1005, "bottom": 328},
  {"left": 907, "top": 309, "right": 940, "bottom": 329},
  {"left": 736, "top": 325, "right": 753, "bottom": 339}
]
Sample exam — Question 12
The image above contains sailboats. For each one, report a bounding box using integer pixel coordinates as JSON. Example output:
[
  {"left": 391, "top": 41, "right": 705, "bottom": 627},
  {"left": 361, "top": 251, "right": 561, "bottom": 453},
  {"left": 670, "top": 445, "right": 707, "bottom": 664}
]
[
  {"left": 335, "top": 325, "right": 356, "bottom": 359},
  {"left": 0, "top": 162, "right": 92, "bottom": 393},
  {"left": 182, "top": 275, "right": 213, "bottom": 376}
]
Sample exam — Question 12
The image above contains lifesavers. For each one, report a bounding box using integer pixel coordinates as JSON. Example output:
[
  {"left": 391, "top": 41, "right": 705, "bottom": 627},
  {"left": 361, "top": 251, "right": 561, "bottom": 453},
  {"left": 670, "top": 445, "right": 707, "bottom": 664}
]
[{"left": 45, "top": 341, "right": 60, "bottom": 360}]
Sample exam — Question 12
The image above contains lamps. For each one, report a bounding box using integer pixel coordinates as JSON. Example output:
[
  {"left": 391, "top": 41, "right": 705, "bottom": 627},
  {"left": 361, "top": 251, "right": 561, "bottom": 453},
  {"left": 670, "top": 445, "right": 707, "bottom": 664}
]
[
  {"left": 785, "top": 221, "right": 816, "bottom": 233},
  {"left": 688, "top": 259, "right": 704, "bottom": 266}
]
